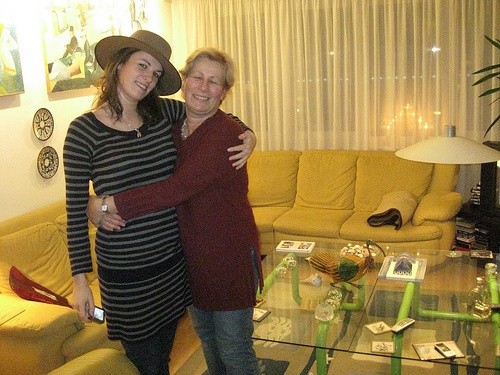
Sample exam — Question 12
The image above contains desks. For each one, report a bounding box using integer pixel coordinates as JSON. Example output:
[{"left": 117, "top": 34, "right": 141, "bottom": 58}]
[{"left": 251, "top": 241, "right": 500, "bottom": 375}]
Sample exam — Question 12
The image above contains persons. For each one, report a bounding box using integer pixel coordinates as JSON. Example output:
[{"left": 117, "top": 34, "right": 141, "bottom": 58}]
[
  {"left": 63, "top": 29, "right": 256, "bottom": 374},
  {"left": 85, "top": 49, "right": 264, "bottom": 375}
]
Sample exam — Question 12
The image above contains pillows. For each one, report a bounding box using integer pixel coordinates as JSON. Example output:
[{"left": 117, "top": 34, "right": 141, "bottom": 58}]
[
  {"left": 411, "top": 191, "right": 463, "bottom": 226},
  {"left": 247, "top": 151, "right": 302, "bottom": 208},
  {"left": 354, "top": 155, "right": 434, "bottom": 212},
  {"left": 55, "top": 213, "right": 98, "bottom": 282},
  {"left": 0, "top": 222, "right": 74, "bottom": 297},
  {"left": 292, "top": 149, "right": 359, "bottom": 210}
]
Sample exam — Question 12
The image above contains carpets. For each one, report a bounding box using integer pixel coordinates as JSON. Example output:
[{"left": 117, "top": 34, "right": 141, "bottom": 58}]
[{"left": 174, "top": 278, "right": 500, "bottom": 375}]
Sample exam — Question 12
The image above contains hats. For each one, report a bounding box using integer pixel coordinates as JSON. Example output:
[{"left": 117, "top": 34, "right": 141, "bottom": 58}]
[{"left": 93, "top": 29, "right": 182, "bottom": 96}]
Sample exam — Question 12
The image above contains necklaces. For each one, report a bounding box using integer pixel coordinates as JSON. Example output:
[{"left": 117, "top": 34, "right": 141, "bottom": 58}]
[
  {"left": 181, "top": 118, "right": 187, "bottom": 138},
  {"left": 123, "top": 116, "right": 142, "bottom": 138}
]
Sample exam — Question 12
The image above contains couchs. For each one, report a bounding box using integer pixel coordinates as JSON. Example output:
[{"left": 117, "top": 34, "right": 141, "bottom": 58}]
[
  {"left": 247, "top": 149, "right": 463, "bottom": 272},
  {"left": 0, "top": 185, "right": 195, "bottom": 375}
]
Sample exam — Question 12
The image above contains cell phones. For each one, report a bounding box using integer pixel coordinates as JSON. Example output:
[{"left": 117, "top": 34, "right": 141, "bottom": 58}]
[{"left": 435, "top": 343, "right": 456, "bottom": 358}]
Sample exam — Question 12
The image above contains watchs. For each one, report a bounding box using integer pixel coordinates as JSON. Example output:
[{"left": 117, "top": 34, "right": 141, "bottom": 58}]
[{"left": 101, "top": 197, "right": 111, "bottom": 214}]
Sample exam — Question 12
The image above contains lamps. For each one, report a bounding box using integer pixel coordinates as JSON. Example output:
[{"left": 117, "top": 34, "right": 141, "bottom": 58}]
[{"left": 395, "top": 0, "right": 500, "bottom": 165}]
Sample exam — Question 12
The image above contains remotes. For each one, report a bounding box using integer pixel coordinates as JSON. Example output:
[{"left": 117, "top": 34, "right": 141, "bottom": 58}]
[{"left": 391, "top": 318, "right": 415, "bottom": 333}]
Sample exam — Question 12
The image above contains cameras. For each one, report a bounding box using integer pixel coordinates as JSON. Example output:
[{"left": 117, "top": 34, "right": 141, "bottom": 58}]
[{"left": 85, "top": 303, "right": 105, "bottom": 323}]
